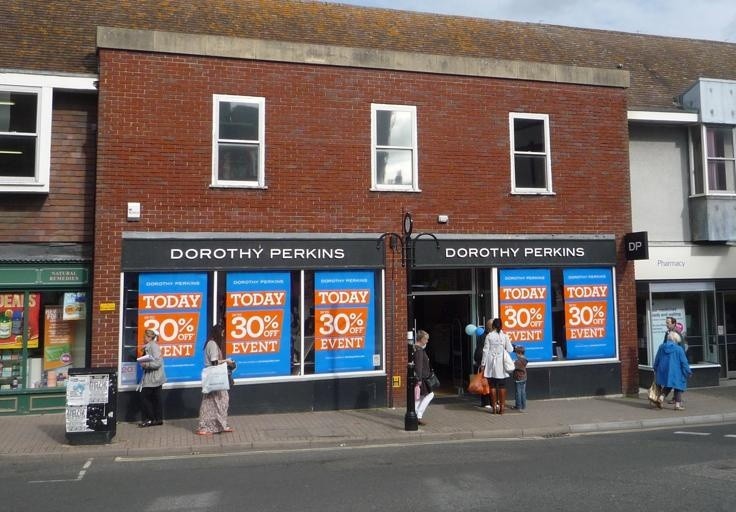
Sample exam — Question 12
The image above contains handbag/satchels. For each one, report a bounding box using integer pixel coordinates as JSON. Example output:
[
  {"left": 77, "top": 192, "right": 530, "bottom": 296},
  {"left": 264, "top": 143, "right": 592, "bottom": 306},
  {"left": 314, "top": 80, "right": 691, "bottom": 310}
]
[
  {"left": 201, "top": 359, "right": 230, "bottom": 395},
  {"left": 467, "top": 369, "right": 489, "bottom": 395},
  {"left": 503, "top": 350, "right": 515, "bottom": 371},
  {"left": 648, "top": 381, "right": 661, "bottom": 403},
  {"left": 424, "top": 371, "right": 441, "bottom": 393}
]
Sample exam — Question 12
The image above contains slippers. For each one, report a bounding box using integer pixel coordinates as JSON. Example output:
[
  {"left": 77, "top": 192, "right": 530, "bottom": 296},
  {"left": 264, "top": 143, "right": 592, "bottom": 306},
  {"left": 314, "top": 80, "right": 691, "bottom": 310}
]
[
  {"left": 196, "top": 431, "right": 210, "bottom": 435},
  {"left": 226, "top": 427, "right": 233, "bottom": 433}
]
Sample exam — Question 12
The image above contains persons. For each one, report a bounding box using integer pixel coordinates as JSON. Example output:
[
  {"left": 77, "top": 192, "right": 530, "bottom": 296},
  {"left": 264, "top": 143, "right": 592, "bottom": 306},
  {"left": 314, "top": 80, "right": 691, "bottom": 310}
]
[
  {"left": 414, "top": 330, "right": 434, "bottom": 425},
  {"left": 138, "top": 330, "right": 165, "bottom": 426},
  {"left": 475, "top": 318, "right": 513, "bottom": 415},
  {"left": 198, "top": 324, "right": 235, "bottom": 435},
  {"left": 513, "top": 346, "right": 528, "bottom": 410},
  {"left": 652, "top": 317, "right": 693, "bottom": 410}
]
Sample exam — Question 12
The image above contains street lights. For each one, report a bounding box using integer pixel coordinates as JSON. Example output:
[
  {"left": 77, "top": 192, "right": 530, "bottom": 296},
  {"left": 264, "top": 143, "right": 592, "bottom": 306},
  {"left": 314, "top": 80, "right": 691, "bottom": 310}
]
[{"left": 376, "top": 213, "right": 440, "bottom": 431}]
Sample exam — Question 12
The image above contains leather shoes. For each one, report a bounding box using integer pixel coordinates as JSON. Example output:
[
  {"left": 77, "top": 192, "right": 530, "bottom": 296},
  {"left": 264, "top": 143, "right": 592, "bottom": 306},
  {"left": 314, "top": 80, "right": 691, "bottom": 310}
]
[{"left": 417, "top": 419, "right": 426, "bottom": 426}]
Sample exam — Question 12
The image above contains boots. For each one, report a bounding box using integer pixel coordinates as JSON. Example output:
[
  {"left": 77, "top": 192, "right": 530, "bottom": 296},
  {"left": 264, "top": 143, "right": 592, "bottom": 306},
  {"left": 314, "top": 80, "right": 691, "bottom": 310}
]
[{"left": 490, "top": 388, "right": 505, "bottom": 414}]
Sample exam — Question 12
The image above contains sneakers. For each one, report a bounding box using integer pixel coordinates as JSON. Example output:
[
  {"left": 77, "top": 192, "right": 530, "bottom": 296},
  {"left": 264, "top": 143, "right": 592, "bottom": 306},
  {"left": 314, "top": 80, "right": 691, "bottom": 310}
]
[
  {"left": 138, "top": 419, "right": 162, "bottom": 427},
  {"left": 656, "top": 398, "right": 685, "bottom": 410},
  {"left": 511, "top": 406, "right": 526, "bottom": 410}
]
